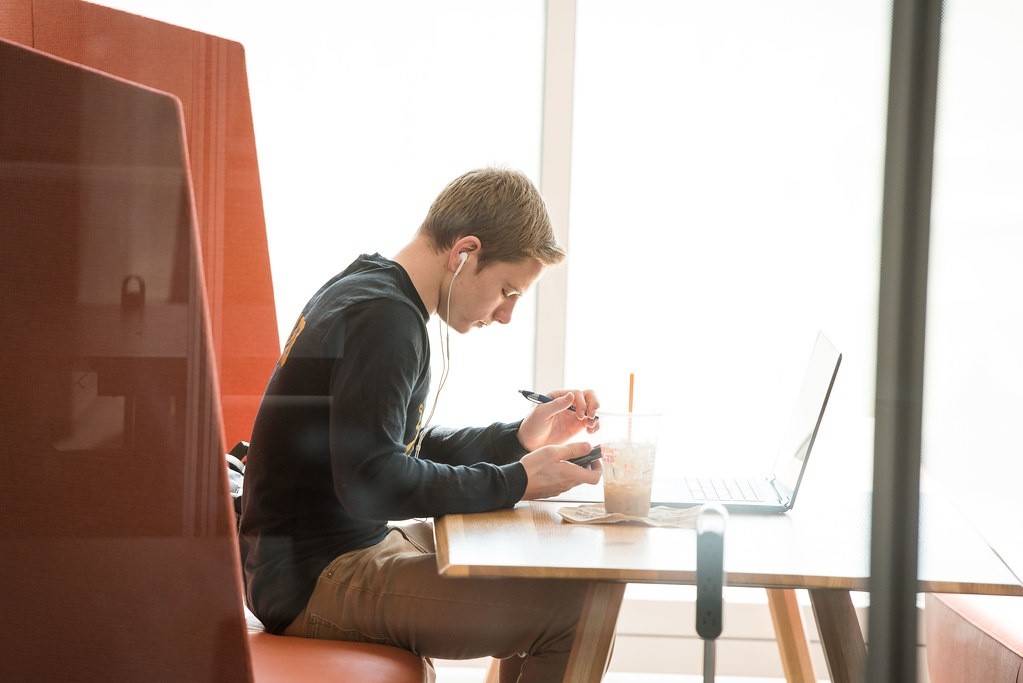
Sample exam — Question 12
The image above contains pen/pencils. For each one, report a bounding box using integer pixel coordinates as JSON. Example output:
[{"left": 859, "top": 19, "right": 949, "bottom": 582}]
[{"left": 518, "top": 388, "right": 601, "bottom": 420}]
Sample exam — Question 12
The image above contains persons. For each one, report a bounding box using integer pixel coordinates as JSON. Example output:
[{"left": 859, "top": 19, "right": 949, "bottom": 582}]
[{"left": 241, "top": 164, "right": 607, "bottom": 683}]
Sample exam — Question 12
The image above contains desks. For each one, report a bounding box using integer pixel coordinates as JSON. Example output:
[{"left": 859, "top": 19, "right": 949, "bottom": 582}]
[{"left": 431, "top": 500, "right": 1023, "bottom": 682}]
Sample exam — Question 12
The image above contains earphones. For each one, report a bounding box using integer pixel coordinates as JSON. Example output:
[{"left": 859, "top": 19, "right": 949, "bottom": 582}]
[{"left": 454, "top": 251, "right": 468, "bottom": 276}]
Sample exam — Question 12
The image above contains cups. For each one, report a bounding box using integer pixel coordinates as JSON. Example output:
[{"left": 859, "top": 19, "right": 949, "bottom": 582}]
[{"left": 595, "top": 409, "right": 662, "bottom": 518}]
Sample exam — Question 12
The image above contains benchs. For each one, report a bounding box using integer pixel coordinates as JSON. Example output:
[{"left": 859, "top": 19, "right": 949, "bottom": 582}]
[{"left": 243, "top": 601, "right": 436, "bottom": 683}]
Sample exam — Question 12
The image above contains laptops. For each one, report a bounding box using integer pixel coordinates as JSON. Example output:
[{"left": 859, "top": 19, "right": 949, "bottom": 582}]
[{"left": 650, "top": 350, "right": 843, "bottom": 513}]
